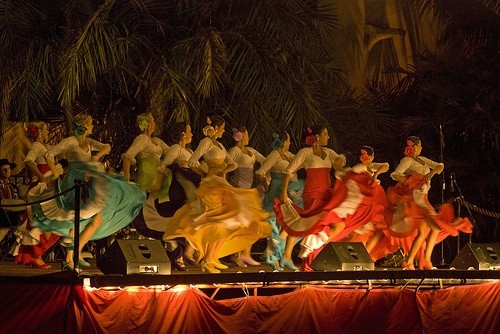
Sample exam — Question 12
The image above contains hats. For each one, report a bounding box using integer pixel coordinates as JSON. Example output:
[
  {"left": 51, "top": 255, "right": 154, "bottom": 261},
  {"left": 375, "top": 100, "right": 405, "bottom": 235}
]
[{"left": 0, "top": 158, "right": 17, "bottom": 169}]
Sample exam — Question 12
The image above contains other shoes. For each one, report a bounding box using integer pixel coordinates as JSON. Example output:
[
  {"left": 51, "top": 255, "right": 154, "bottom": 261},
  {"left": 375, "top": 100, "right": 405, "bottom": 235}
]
[
  {"left": 240, "top": 254, "right": 261, "bottom": 266},
  {"left": 230, "top": 254, "right": 248, "bottom": 268},
  {"left": 5, "top": 253, "right": 15, "bottom": 261}
]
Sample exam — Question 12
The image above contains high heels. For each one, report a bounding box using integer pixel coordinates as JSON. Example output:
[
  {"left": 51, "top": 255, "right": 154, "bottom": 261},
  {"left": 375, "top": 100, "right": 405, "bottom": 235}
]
[
  {"left": 201, "top": 260, "right": 221, "bottom": 274},
  {"left": 72, "top": 252, "right": 91, "bottom": 267},
  {"left": 183, "top": 253, "right": 196, "bottom": 266},
  {"left": 268, "top": 263, "right": 284, "bottom": 271},
  {"left": 213, "top": 261, "right": 229, "bottom": 270},
  {"left": 418, "top": 258, "right": 433, "bottom": 270},
  {"left": 60, "top": 259, "right": 83, "bottom": 273},
  {"left": 29, "top": 258, "right": 52, "bottom": 269},
  {"left": 171, "top": 260, "right": 189, "bottom": 271},
  {"left": 401, "top": 261, "right": 416, "bottom": 270},
  {"left": 280, "top": 257, "right": 299, "bottom": 270}
]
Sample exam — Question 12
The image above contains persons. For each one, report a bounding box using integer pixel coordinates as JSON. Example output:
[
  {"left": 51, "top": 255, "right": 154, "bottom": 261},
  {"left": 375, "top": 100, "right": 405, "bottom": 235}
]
[
  {"left": 384, "top": 136, "right": 473, "bottom": 270},
  {"left": 162, "top": 115, "right": 276, "bottom": 274},
  {"left": 26, "top": 113, "right": 146, "bottom": 274},
  {"left": 0, "top": 112, "right": 387, "bottom": 271},
  {"left": 273, "top": 124, "right": 380, "bottom": 272}
]
